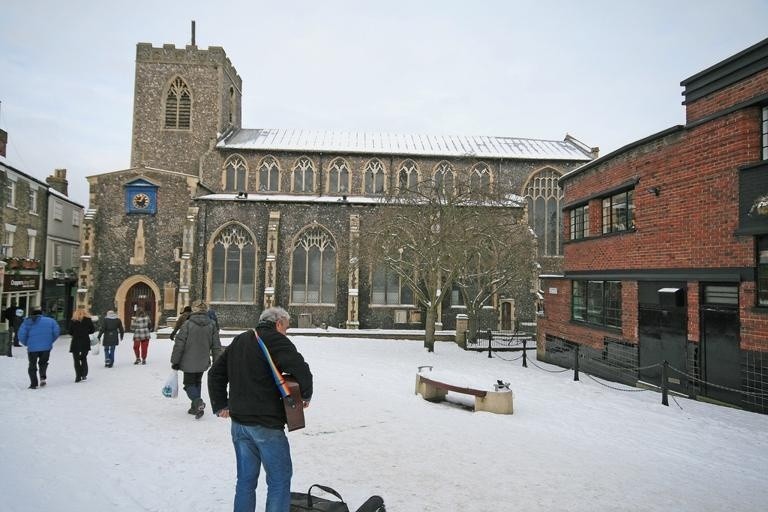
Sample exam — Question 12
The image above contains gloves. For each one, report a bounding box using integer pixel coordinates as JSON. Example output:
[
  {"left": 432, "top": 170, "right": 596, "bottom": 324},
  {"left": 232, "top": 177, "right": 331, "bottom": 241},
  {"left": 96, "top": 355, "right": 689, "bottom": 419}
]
[{"left": 170, "top": 333, "right": 174, "bottom": 340}]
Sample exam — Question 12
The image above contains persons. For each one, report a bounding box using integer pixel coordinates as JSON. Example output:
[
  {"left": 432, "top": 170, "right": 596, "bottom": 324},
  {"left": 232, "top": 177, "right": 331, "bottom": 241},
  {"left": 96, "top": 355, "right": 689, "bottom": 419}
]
[
  {"left": 67, "top": 308, "right": 95, "bottom": 383},
  {"left": 207, "top": 305, "right": 314, "bottom": 512},
  {"left": 170, "top": 298, "right": 223, "bottom": 419},
  {"left": 12, "top": 307, "right": 24, "bottom": 347},
  {"left": 204, "top": 302, "right": 220, "bottom": 331},
  {"left": 130, "top": 307, "right": 154, "bottom": 365},
  {"left": 169, "top": 305, "right": 193, "bottom": 340},
  {"left": 17, "top": 309, "right": 62, "bottom": 389},
  {"left": 97, "top": 310, "right": 124, "bottom": 367}
]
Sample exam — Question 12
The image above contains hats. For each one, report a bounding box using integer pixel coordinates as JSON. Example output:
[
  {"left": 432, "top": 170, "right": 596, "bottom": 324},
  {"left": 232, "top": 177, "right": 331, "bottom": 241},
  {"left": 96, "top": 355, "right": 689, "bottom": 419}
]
[{"left": 180, "top": 306, "right": 191, "bottom": 313}]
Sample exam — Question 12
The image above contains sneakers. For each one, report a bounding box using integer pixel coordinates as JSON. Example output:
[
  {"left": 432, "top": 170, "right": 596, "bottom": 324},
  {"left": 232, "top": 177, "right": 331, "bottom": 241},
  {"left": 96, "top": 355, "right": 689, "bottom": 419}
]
[
  {"left": 188, "top": 399, "right": 205, "bottom": 419},
  {"left": 134, "top": 359, "right": 141, "bottom": 364},
  {"left": 40, "top": 380, "right": 46, "bottom": 386},
  {"left": 142, "top": 361, "right": 146, "bottom": 365}
]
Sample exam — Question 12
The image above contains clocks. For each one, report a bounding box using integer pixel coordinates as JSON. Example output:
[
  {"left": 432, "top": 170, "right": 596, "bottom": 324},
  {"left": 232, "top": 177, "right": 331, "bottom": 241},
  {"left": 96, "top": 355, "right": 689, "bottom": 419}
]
[{"left": 133, "top": 193, "right": 150, "bottom": 209}]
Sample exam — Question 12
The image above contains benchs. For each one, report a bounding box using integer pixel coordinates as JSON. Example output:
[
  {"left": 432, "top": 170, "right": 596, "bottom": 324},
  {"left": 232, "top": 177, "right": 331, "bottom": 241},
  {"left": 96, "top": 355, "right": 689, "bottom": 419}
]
[{"left": 414, "top": 364, "right": 513, "bottom": 417}]
